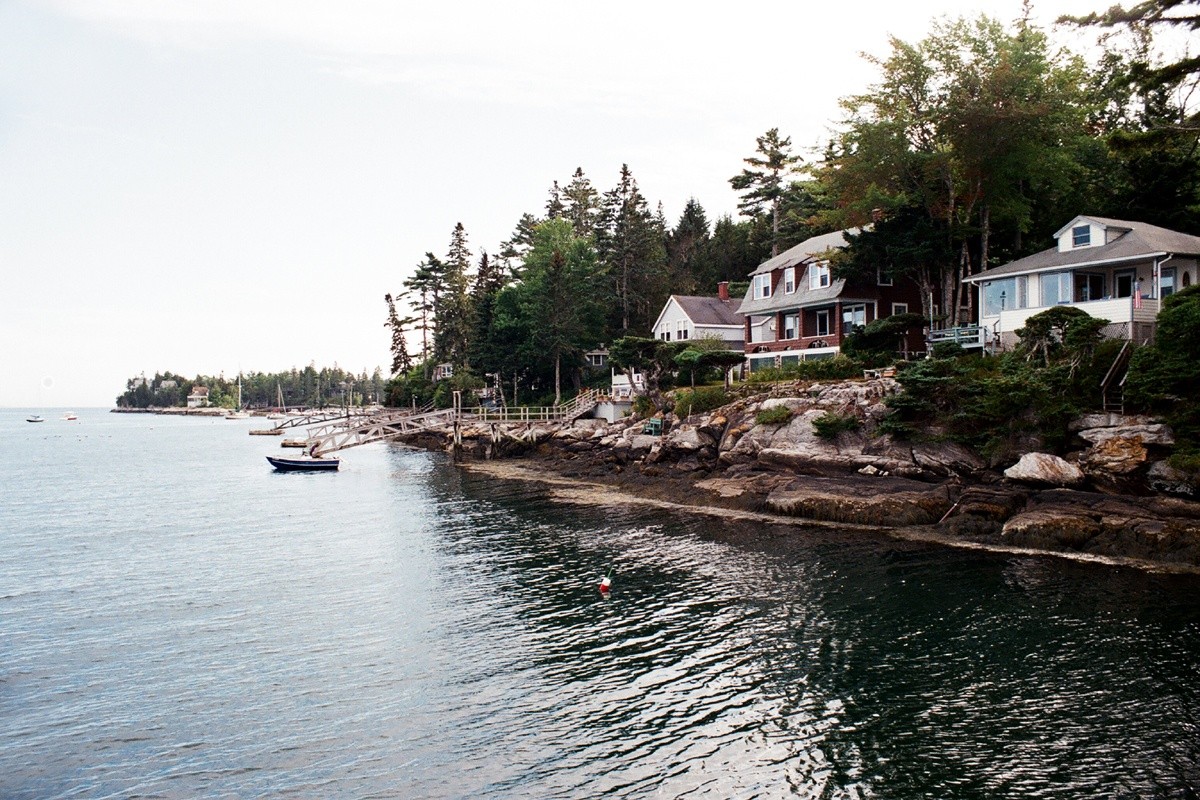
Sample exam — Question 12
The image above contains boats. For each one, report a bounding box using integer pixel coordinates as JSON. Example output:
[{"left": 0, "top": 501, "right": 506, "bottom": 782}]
[
  {"left": 67, "top": 416, "right": 78, "bottom": 421},
  {"left": 265, "top": 440, "right": 340, "bottom": 473},
  {"left": 25, "top": 415, "right": 44, "bottom": 422}
]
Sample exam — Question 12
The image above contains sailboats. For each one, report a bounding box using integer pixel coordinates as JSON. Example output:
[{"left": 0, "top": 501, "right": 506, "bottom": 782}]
[
  {"left": 224, "top": 375, "right": 250, "bottom": 419},
  {"left": 287, "top": 390, "right": 380, "bottom": 417},
  {"left": 267, "top": 383, "right": 288, "bottom": 420}
]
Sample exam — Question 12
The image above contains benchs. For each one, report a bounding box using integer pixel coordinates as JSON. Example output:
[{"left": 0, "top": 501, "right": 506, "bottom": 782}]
[{"left": 644, "top": 417, "right": 664, "bottom": 436}]
